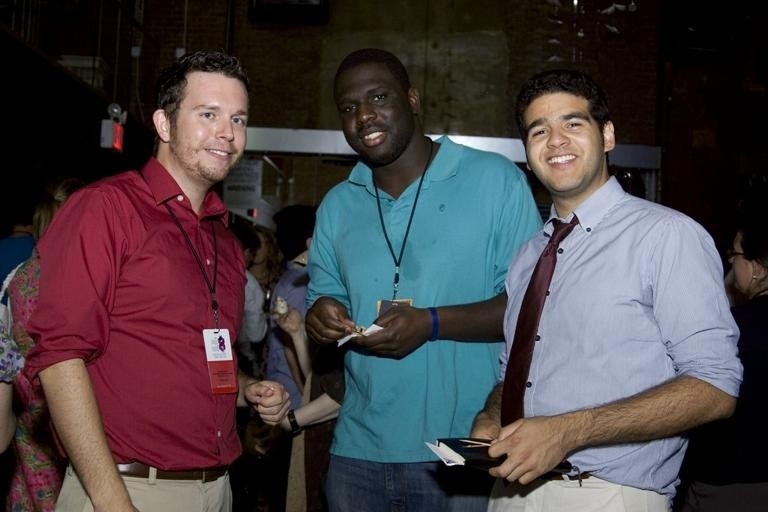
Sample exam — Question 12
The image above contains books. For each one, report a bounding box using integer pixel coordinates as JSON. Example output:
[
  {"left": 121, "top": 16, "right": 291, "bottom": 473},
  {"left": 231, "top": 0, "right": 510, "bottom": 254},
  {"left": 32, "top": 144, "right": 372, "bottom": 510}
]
[{"left": 433, "top": 438, "right": 573, "bottom": 473}]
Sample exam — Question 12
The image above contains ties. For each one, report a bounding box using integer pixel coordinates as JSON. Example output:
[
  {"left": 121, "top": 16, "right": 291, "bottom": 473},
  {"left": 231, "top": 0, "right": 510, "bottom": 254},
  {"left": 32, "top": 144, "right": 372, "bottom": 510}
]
[{"left": 501, "top": 214, "right": 577, "bottom": 428}]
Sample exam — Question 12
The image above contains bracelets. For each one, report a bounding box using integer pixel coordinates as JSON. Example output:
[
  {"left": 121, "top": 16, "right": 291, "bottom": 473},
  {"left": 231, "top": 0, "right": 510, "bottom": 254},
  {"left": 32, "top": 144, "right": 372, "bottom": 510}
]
[{"left": 427, "top": 307, "right": 438, "bottom": 344}]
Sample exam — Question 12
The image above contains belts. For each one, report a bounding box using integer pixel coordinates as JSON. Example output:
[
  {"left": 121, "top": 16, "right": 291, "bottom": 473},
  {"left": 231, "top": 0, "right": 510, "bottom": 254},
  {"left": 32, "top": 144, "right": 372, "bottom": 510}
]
[{"left": 115, "top": 462, "right": 227, "bottom": 481}]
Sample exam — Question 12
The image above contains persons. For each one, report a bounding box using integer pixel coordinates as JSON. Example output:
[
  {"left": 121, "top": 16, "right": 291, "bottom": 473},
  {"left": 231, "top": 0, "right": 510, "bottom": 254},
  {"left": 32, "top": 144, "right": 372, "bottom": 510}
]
[
  {"left": 302, "top": 46, "right": 544, "bottom": 511},
  {"left": 1, "top": 175, "right": 346, "bottom": 512},
  {"left": 465, "top": 68, "right": 745, "bottom": 511},
  {"left": 671, "top": 216, "right": 766, "bottom": 511},
  {"left": 29, "top": 43, "right": 292, "bottom": 511}
]
